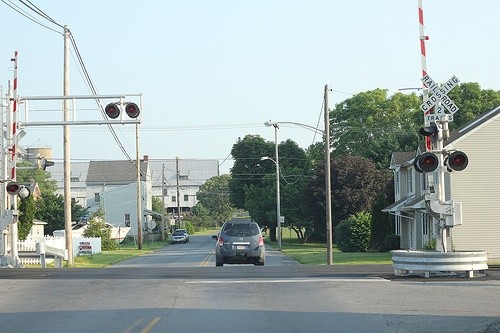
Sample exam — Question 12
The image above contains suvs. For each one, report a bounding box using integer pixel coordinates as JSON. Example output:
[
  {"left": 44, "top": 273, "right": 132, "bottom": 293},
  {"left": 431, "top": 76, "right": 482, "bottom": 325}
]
[{"left": 213, "top": 220, "right": 266, "bottom": 266}]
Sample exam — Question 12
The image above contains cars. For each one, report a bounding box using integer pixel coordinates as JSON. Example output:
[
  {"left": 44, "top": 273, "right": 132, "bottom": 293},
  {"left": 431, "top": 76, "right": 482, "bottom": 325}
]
[{"left": 172, "top": 229, "right": 189, "bottom": 244}]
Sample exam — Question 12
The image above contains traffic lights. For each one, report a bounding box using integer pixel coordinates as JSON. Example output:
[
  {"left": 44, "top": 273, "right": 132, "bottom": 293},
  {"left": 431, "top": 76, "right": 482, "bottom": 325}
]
[
  {"left": 125, "top": 103, "right": 139, "bottom": 118},
  {"left": 43, "top": 159, "right": 54, "bottom": 170},
  {"left": 412, "top": 152, "right": 440, "bottom": 173},
  {"left": 6, "top": 182, "right": 20, "bottom": 194},
  {"left": 443, "top": 151, "right": 469, "bottom": 173},
  {"left": 419, "top": 122, "right": 443, "bottom": 142},
  {"left": 105, "top": 103, "right": 120, "bottom": 118}
]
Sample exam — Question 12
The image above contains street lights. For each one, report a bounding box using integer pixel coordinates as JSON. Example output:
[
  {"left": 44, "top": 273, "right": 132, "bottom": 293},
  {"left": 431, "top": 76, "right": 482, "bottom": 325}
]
[
  {"left": 264, "top": 120, "right": 332, "bottom": 265},
  {"left": 261, "top": 156, "right": 282, "bottom": 248}
]
